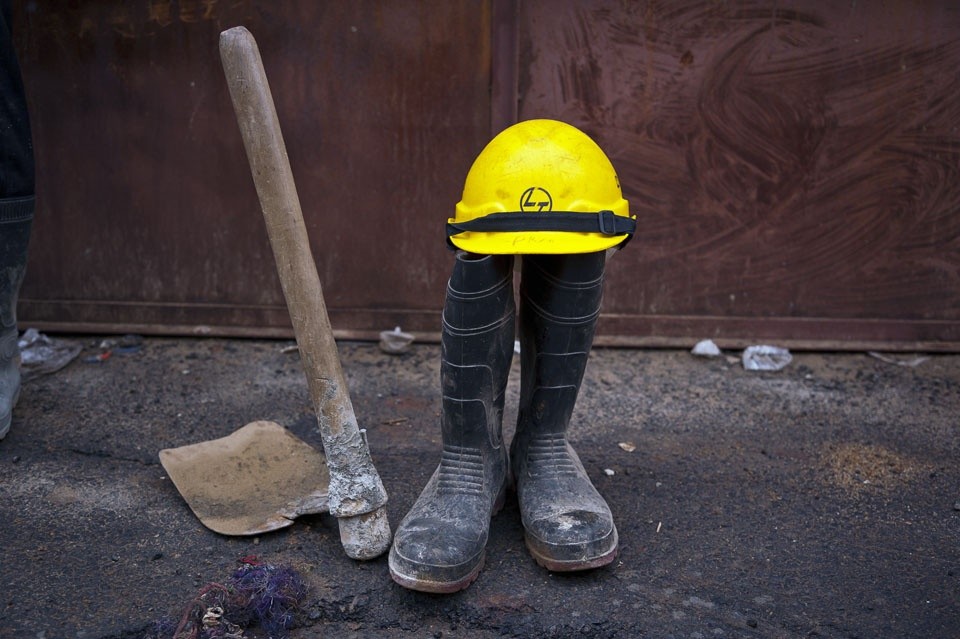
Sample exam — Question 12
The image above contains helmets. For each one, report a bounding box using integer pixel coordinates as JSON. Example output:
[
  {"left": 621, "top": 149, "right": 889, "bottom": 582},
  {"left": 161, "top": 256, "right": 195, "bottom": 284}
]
[{"left": 445, "top": 118, "right": 637, "bottom": 254}]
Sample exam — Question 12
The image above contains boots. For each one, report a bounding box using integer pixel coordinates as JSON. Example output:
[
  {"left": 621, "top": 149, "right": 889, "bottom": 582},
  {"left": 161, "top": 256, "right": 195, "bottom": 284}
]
[
  {"left": 389, "top": 253, "right": 517, "bottom": 593},
  {"left": 509, "top": 250, "right": 618, "bottom": 571},
  {"left": 0, "top": 196, "right": 34, "bottom": 440}
]
